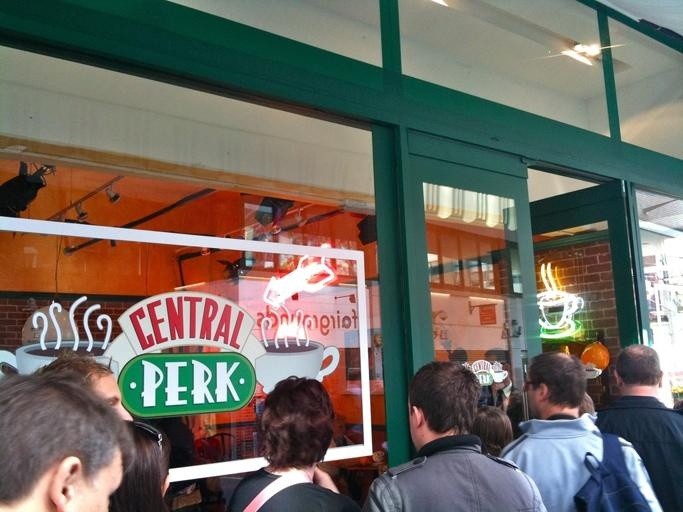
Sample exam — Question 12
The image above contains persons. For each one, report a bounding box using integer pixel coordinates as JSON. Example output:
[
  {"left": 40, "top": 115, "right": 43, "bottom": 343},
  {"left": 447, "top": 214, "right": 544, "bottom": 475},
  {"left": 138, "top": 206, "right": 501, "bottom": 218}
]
[{"left": 1, "top": 341, "right": 683, "bottom": 512}]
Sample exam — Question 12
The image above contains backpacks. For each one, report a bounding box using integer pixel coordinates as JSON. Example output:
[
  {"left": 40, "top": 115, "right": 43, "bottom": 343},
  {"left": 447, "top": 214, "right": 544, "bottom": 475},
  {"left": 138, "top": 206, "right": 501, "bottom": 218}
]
[{"left": 574, "top": 432, "right": 653, "bottom": 512}]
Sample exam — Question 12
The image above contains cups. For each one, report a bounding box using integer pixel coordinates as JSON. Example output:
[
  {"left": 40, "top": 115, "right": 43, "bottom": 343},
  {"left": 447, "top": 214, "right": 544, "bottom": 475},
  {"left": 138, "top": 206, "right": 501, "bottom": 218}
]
[
  {"left": 254, "top": 339, "right": 340, "bottom": 394},
  {"left": 474, "top": 369, "right": 508, "bottom": 384},
  {"left": 585, "top": 367, "right": 602, "bottom": 378},
  {"left": 0, "top": 341, "right": 111, "bottom": 376}
]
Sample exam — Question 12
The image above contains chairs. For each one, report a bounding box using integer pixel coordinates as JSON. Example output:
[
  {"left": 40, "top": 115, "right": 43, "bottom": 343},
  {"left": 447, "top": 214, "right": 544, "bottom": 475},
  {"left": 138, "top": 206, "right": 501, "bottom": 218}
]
[
  {"left": 207, "top": 431, "right": 242, "bottom": 512},
  {"left": 165, "top": 433, "right": 203, "bottom": 511}
]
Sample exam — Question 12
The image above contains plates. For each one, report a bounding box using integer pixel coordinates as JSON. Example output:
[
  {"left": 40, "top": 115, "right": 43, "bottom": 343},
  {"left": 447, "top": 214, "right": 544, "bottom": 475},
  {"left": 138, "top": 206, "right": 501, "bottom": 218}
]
[{"left": 539, "top": 319, "right": 582, "bottom": 341}]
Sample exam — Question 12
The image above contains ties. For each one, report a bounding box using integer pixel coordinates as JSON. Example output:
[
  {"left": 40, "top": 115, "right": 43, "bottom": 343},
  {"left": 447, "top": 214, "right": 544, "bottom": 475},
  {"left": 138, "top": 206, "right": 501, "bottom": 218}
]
[{"left": 495, "top": 390, "right": 504, "bottom": 410}]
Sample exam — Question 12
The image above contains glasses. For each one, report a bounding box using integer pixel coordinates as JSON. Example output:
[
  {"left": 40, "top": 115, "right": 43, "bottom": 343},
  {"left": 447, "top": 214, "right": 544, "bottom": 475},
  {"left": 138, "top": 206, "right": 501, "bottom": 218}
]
[
  {"left": 133, "top": 420, "right": 163, "bottom": 455},
  {"left": 524, "top": 382, "right": 532, "bottom": 391}
]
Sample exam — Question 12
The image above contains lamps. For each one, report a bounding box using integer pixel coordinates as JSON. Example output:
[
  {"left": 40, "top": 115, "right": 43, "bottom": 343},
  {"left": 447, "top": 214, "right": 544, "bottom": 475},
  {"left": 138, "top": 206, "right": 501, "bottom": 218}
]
[
  {"left": 218, "top": 251, "right": 255, "bottom": 286},
  {"left": 356, "top": 215, "right": 378, "bottom": 246},
  {"left": 41, "top": 178, "right": 118, "bottom": 225},
  {"left": 254, "top": 197, "right": 292, "bottom": 224},
  {"left": 0, "top": 158, "right": 55, "bottom": 238}
]
